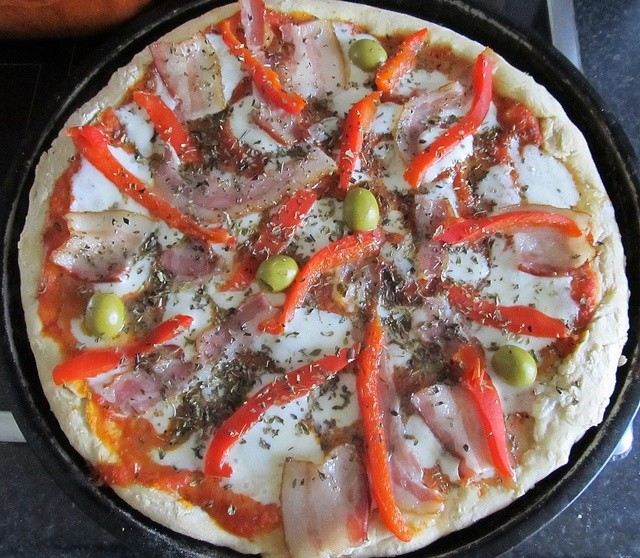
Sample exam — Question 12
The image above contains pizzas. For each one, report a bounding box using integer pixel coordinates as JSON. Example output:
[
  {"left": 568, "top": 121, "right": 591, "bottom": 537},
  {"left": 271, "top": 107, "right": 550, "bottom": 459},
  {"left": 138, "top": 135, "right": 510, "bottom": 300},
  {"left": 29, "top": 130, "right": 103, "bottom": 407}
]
[{"left": 17, "top": 1, "right": 629, "bottom": 556}]
